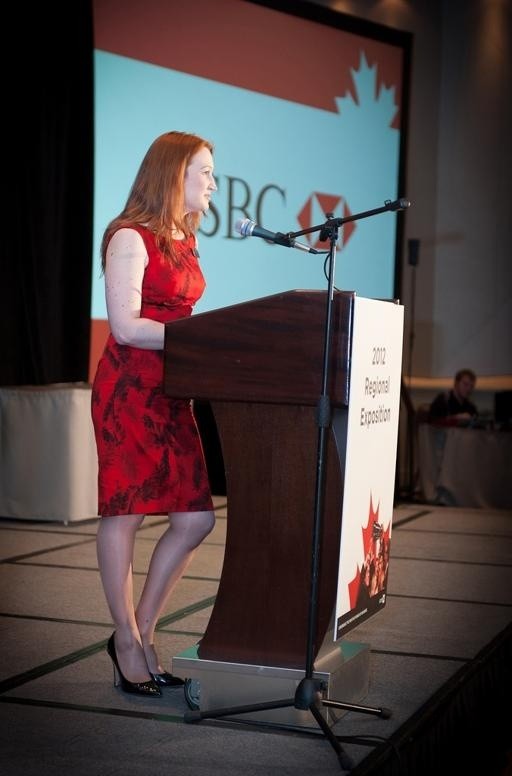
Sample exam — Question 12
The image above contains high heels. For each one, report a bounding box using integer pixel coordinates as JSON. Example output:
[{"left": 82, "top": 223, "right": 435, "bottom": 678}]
[
  {"left": 108, "top": 631, "right": 162, "bottom": 699},
  {"left": 150, "top": 671, "right": 185, "bottom": 687}
]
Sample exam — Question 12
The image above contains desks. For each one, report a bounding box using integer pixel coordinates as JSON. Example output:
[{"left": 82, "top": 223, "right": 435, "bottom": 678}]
[{"left": 413, "top": 423, "right": 512, "bottom": 510}]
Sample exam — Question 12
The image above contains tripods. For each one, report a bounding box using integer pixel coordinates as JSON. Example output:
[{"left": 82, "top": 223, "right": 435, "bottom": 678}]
[{"left": 181, "top": 233, "right": 410, "bottom": 771}]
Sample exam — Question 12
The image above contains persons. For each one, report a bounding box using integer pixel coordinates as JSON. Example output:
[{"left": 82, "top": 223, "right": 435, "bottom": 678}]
[
  {"left": 428, "top": 370, "right": 479, "bottom": 427},
  {"left": 91, "top": 131, "right": 217, "bottom": 699},
  {"left": 361, "top": 540, "right": 389, "bottom": 597}
]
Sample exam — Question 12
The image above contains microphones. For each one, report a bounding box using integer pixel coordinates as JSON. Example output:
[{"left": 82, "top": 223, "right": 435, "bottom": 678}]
[{"left": 236, "top": 218, "right": 317, "bottom": 256}]
